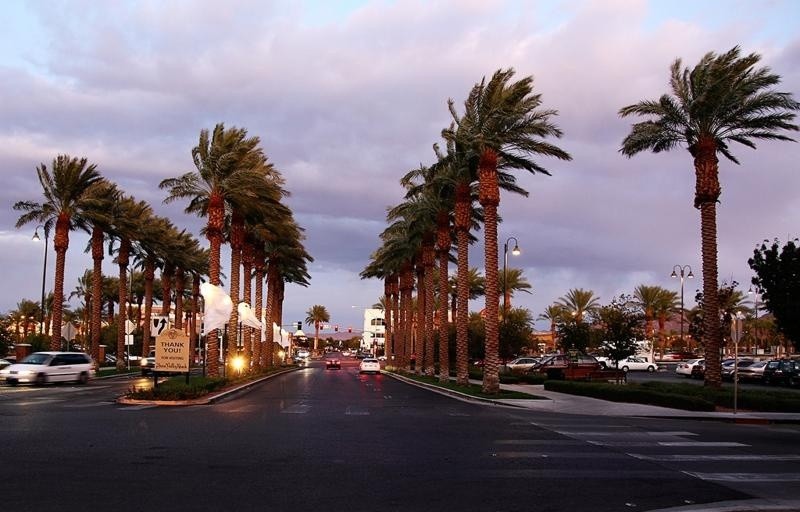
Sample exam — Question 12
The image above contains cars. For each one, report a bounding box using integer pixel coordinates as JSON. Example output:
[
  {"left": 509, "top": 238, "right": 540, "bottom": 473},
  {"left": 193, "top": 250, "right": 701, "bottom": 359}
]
[
  {"left": 506, "top": 357, "right": 540, "bottom": 371},
  {"left": 354, "top": 353, "right": 380, "bottom": 374},
  {"left": 676, "top": 359, "right": 782, "bottom": 382},
  {"left": 379, "top": 355, "right": 394, "bottom": 361},
  {"left": 673, "top": 352, "right": 697, "bottom": 359},
  {"left": 597, "top": 356, "right": 658, "bottom": 372}
]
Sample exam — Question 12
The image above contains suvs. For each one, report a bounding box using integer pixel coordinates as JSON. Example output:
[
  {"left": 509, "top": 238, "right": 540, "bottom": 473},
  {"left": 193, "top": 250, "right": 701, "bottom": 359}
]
[{"left": 140, "top": 351, "right": 155, "bottom": 374}]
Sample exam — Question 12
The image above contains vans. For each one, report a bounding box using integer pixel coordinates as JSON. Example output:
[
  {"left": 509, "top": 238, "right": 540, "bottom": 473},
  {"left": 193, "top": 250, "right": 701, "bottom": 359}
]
[
  {"left": 326, "top": 352, "right": 341, "bottom": 369},
  {"left": 0, "top": 352, "right": 96, "bottom": 387}
]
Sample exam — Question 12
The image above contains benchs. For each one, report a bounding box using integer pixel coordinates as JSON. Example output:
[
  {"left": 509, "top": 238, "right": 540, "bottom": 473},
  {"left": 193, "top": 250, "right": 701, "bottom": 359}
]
[{"left": 587, "top": 365, "right": 629, "bottom": 384}]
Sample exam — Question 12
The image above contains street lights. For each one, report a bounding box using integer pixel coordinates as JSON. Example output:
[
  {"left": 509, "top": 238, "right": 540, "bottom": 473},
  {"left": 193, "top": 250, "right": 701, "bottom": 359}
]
[
  {"left": 671, "top": 264, "right": 695, "bottom": 360},
  {"left": 749, "top": 284, "right": 764, "bottom": 356},
  {"left": 504, "top": 237, "right": 521, "bottom": 371},
  {"left": 32, "top": 225, "right": 48, "bottom": 334}
]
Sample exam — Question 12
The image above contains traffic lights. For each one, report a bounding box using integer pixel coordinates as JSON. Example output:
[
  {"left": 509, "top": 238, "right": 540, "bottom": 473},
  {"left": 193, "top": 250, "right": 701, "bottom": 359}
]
[
  {"left": 335, "top": 325, "right": 338, "bottom": 331},
  {"left": 349, "top": 328, "right": 352, "bottom": 333},
  {"left": 320, "top": 324, "right": 323, "bottom": 330}
]
